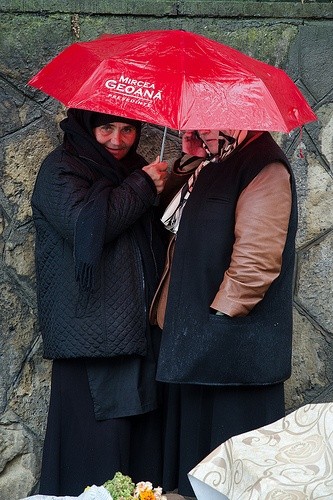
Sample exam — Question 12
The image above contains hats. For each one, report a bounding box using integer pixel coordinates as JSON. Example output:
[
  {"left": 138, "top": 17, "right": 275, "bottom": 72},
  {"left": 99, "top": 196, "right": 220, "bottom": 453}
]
[{"left": 90, "top": 111, "right": 141, "bottom": 140}]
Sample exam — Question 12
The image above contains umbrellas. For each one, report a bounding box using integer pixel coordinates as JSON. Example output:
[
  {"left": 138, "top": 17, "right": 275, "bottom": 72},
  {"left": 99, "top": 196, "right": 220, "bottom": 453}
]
[{"left": 26, "top": 30, "right": 317, "bottom": 161}]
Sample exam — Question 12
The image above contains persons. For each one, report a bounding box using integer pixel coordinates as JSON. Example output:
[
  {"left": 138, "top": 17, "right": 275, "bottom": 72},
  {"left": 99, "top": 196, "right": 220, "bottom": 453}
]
[
  {"left": 30, "top": 108, "right": 168, "bottom": 498},
  {"left": 149, "top": 130, "right": 298, "bottom": 500}
]
